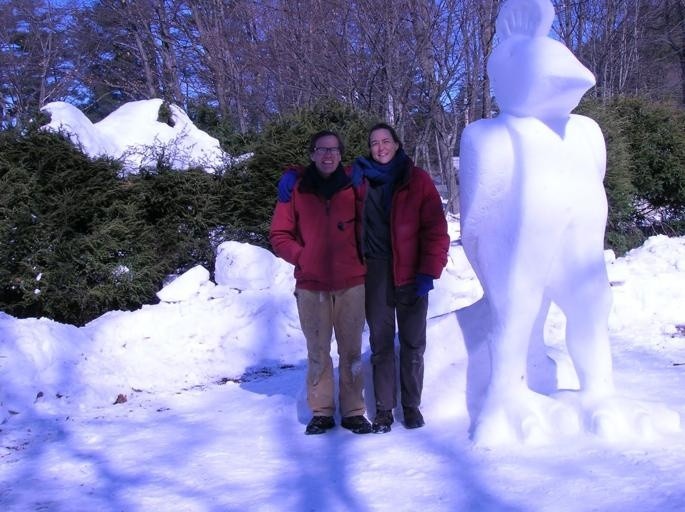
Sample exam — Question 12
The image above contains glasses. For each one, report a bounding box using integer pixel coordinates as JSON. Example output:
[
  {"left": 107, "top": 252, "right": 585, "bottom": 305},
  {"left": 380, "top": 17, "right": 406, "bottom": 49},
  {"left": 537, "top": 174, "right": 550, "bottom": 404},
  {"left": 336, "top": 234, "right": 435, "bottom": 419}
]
[{"left": 315, "top": 147, "right": 339, "bottom": 154}]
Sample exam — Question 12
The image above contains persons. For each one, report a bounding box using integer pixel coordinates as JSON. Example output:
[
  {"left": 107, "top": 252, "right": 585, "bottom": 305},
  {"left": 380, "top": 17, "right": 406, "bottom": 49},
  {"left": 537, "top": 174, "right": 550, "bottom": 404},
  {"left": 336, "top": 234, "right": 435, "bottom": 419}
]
[
  {"left": 276, "top": 122, "right": 450, "bottom": 435},
  {"left": 269, "top": 131, "right": 376, "bottom": 432}
]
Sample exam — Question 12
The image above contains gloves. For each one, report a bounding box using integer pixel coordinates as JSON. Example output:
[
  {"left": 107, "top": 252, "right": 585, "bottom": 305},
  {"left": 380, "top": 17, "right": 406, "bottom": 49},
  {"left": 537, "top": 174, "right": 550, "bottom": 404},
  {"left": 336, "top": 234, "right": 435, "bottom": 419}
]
[
  {"left": 416, "top": 274, "right": 433, "bottom": 296},
  {"left": 278, "top": 170, "right": 300, "bottom": 202}
]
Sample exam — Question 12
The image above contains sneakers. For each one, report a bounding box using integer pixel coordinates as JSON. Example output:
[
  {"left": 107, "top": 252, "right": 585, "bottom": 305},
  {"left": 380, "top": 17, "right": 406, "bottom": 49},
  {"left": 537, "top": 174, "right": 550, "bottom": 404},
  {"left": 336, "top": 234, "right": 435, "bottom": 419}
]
[
  {"left": 403, "top": 406, "right": 423, "bottom": 427},
  {"left": 307, "top": 416, "right": 334, "bottom": 433},
  {"left": 341, "top": 415, "right": 371, "bottom": 434},
  {"left": 372, "top": 409, "right": 393, "bottom": 433}
]
